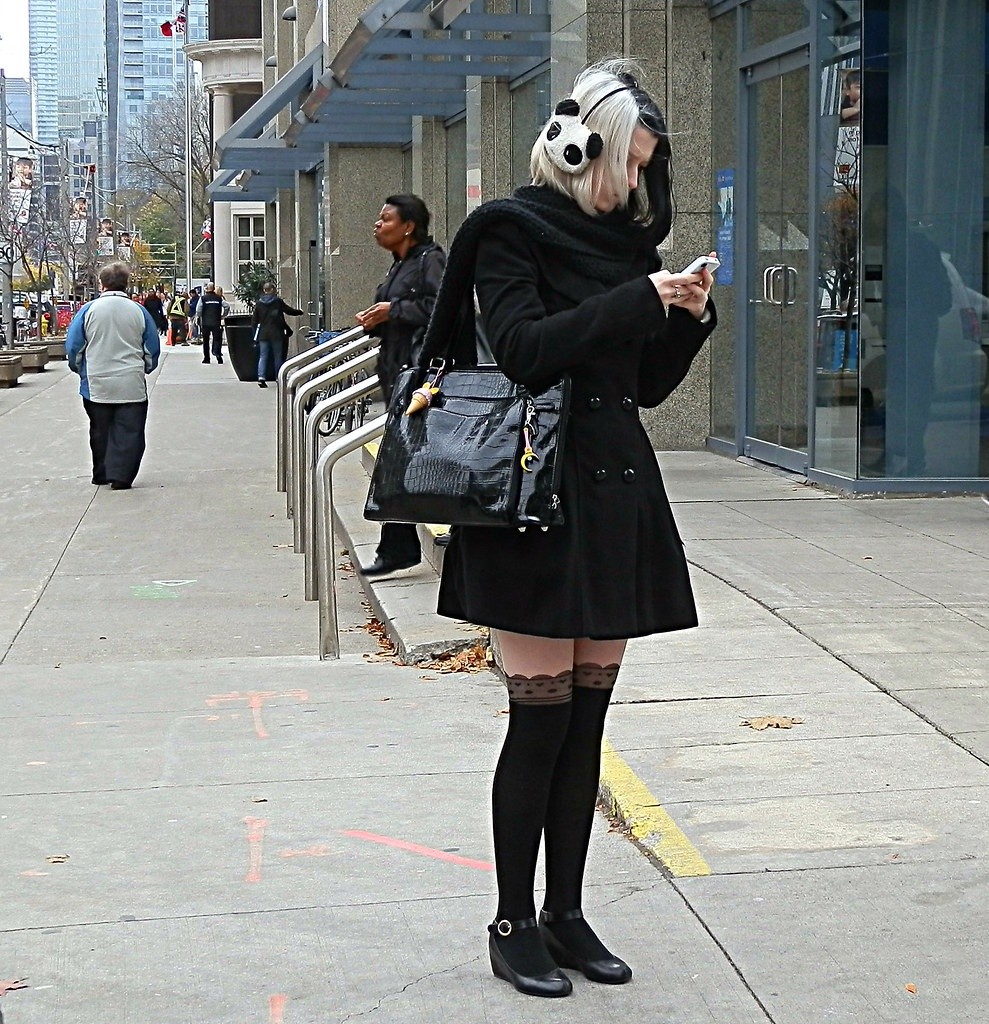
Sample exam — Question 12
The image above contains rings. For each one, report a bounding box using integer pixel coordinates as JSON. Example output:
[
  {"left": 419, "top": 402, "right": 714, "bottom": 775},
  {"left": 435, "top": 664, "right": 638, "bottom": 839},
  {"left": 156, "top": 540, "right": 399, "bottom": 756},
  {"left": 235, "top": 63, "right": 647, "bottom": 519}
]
[{"left": 674, "top": 286, "right": 681, "bottom": 298}]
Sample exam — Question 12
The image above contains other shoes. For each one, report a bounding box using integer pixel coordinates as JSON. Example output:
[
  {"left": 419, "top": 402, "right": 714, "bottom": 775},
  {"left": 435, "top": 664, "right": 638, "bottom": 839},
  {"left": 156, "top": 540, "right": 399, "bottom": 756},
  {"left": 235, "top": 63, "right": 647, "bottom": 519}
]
[
  {"left": 181, "top": 343, "right": 190, "bottom": 346},
  {"left": 202, "top": 357, "right": 210, "bottom": 363},
  {"left": 111, "top": 481, "right": 131, "bottom": 489},
  {"left": 258, "top": 380, "right": 267, "bottom": 388},
  {"left": 217, "top": 356, "right": 223, "bottom": 364}
]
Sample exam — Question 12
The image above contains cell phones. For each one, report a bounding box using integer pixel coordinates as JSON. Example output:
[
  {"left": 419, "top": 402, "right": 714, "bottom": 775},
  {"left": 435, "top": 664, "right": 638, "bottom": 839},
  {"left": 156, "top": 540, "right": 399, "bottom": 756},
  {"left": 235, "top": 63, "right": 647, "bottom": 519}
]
[{"left": 675, "top": 256, "right": 721, "bottom": 287}]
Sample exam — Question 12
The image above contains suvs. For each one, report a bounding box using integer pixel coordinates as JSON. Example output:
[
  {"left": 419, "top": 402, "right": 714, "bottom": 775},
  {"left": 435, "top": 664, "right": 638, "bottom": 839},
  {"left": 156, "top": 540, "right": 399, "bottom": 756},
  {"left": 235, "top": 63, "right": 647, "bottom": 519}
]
[{"left": 0, "top": 291, "right": 81, "bottom": 346}]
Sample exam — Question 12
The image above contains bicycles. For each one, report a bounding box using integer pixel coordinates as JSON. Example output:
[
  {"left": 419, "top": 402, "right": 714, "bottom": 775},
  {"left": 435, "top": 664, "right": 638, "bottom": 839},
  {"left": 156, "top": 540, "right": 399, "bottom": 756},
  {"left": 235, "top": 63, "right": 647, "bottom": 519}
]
[{"left": 300, "top": 326, "right": 370, "bottom": 436}]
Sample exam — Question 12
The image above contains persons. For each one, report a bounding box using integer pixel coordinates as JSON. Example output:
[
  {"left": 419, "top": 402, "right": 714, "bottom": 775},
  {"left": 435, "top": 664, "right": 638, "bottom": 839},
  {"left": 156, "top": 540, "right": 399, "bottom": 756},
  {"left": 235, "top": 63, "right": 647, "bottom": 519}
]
[
  {"left": 99, "top": 219, "right": 113, "bottom": 236},
  {"left": 70, "top": 197, "right": 87, "bottom": 218},
  {"left": 64, "top": 262, "right": 160, "bottom": 489},
  {"left": 11, "top": 157, "right": 33, "bottom": 186},
  {"left": 353, "top": 192, "right": 448, "bottom": 577},
  {"left": 118, "top": 233, "right": 130, "bottom": 247},
  {"left": 863, "top": 186, "right": 952, "bottom": 478},
  {"left": 432, "top": 58, "right": 720, "bottom": 998},
  {"left": 251, "top": 285, "right": 305, "bottom": 387},
  {"left": 129, "top": 282, "right": 231, "bottom": 364}
]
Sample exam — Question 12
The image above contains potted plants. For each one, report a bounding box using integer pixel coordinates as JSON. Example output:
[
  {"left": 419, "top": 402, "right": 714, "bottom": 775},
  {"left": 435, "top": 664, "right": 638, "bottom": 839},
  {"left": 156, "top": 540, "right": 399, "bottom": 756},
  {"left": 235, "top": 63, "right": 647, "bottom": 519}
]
[{"left": 223, "top": 257, "right": 290, "bottom": 382}]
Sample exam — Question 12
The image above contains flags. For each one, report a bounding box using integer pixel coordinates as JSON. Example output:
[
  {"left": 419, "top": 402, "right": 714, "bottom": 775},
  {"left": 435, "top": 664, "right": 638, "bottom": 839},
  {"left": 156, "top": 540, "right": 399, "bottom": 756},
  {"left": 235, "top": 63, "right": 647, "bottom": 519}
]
[
  {"left": 161, "top": 21, "right": 172, "bottom": 36},
  {"left": 172, "top": 5, "right": 185, "bottom": 33}
]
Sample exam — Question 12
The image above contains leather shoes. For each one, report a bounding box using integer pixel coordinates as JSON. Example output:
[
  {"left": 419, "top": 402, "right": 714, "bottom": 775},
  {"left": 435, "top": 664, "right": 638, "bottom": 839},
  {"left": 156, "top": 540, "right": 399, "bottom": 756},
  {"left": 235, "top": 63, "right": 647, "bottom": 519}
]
[
  {"left": 488, "top": 921, "right": 572, "bottom": 998},
  {"left": 435, "top": 537, "right": 450, "bottom": 546},
  {"left": 539, "top": 909, "right": 633, "bottom": 984},
  {"left": 360, "top": 537, "right": 420, "bottom": 575}
]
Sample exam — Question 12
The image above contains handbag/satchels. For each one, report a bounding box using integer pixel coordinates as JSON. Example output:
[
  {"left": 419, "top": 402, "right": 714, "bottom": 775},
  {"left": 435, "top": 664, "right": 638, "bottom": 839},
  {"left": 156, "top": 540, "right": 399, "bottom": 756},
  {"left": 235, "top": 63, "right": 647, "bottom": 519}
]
[{"left": 362, "top": 283, "right": 572, "bottom": 534}]
